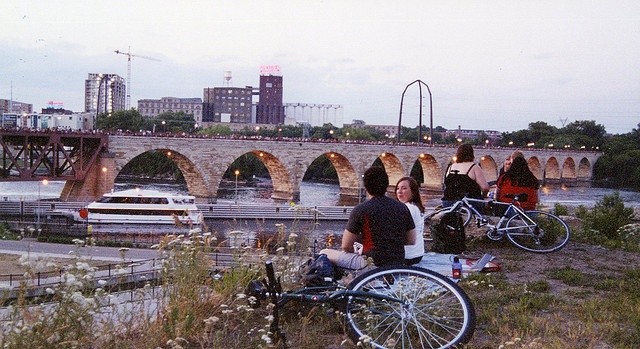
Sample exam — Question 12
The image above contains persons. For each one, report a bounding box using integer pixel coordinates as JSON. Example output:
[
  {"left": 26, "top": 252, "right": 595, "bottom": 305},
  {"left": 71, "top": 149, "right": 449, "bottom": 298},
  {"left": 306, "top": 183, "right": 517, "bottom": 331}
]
[
  {"left": 510, "top": 151, "right": 524, "bottom": 163},
  {"left": 497, "top": 156, "right": 540, "bottom": 211},
  {"left": 441, "top": 144, "right": 491, "bottom": 215},
  {"left": 319, "top": 166, "right": 417, "bottom": 280},
  {"left": 499, "top": 156, "right": 511, "bottom": 177},
  {"left": 395, "top": 177, "right": 425, "bottom": 265}
]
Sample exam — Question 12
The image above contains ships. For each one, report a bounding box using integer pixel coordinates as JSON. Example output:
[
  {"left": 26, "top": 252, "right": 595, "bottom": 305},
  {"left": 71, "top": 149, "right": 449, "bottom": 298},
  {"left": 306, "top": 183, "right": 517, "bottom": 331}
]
[{"left": 74, "top": 190, "right": 203, "bottom": 226}]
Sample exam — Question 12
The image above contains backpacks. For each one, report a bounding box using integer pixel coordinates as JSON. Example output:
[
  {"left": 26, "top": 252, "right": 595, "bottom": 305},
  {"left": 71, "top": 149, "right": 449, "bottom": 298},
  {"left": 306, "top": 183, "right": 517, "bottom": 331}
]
[{"left": 430, "top": 201, "right": 465, "bottom": 254}]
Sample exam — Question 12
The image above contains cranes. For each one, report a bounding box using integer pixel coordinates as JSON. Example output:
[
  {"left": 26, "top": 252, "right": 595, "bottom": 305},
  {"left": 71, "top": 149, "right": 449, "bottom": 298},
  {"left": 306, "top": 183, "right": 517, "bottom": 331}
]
[{"left": 116, "top": 47, "right": 158, "bottom": 109}]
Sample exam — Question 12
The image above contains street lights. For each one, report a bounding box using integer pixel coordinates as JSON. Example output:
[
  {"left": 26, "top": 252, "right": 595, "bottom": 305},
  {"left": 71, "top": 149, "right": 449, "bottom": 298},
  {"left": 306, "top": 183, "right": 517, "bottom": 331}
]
[
  {"left": 235, "top": 169, "right": 239, "bottom": 204},
  {"left": 37, "top": 180, "right": 48, "bottom": 225},
  {"left": 102, "top": 167, "right": 108, "bottom": 193}
]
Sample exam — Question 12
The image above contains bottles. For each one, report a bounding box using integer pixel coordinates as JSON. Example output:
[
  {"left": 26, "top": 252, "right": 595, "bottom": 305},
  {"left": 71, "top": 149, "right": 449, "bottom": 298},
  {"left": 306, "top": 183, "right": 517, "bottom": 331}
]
[{"left": 449, "top": 255, "right": 463, "bottom": 279}]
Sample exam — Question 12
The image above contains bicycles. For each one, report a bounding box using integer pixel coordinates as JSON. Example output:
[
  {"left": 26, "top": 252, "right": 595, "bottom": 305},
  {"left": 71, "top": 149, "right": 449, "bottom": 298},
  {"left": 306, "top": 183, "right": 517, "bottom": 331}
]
[
  {"left": 246, "top": 261, "right": 475, "bottom": 349},
  {"left": 425, "top": 192, "right": 570, "bottom": 252}
]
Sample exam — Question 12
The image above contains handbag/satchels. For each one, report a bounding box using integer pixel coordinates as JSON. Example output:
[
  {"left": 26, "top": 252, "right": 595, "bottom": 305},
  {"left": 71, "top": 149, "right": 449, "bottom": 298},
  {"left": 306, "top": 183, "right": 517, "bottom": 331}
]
[{"left": 297, "top": 254, "right": 343, "bottom": 291}]
